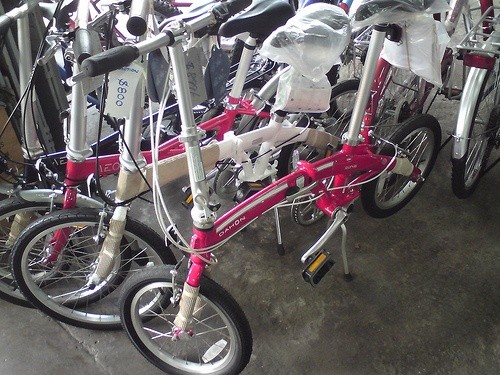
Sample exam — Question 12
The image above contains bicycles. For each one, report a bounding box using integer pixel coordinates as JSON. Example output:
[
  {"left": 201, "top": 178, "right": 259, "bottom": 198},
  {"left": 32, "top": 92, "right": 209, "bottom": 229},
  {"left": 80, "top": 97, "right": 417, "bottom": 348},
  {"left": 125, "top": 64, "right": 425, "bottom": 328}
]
[
  {"left": 0, "top": 0, "right": 280, "bottom": 286},
  {"left": 446, "top": 1, "right": 500, "bottom": 197},
  {"left": 81, "top": 0, "right": 441, "bottom": 375},
  {"left": 0, "top": 0, "right": 338, "bottom": 311},
  {"left": 358, "top": 0, "right": 494, "bottom": 154},
  {"left": 11, "top": 0, "right": 360, "bottom": 331}
]
